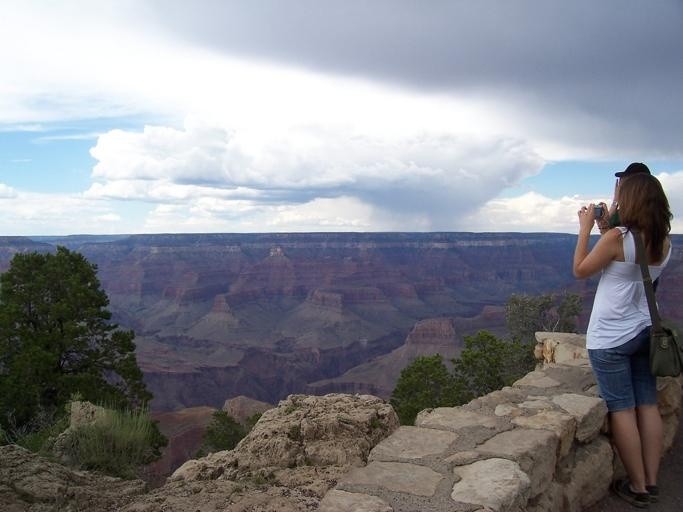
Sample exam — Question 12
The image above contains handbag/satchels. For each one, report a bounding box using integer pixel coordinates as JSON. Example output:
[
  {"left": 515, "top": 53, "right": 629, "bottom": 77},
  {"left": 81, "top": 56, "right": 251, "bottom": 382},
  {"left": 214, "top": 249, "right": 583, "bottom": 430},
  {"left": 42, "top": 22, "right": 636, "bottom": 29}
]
[{"left": 649, "top": 319, "right": 682, "bottom": 378}]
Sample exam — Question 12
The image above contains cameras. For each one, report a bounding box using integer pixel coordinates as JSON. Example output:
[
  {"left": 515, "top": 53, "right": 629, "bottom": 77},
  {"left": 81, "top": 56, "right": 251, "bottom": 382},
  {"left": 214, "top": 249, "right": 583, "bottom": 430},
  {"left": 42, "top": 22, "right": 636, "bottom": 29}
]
[{"left": 593, "top": 206, "right": 602, "bottom": 218}]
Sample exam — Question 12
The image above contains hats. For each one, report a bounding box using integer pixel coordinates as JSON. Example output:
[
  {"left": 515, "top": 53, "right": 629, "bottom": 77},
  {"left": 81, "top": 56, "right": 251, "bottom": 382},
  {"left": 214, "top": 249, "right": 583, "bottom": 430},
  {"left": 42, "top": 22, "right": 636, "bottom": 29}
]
[{"left": 615, "top": 162, "right": 650, "bottom": 177}]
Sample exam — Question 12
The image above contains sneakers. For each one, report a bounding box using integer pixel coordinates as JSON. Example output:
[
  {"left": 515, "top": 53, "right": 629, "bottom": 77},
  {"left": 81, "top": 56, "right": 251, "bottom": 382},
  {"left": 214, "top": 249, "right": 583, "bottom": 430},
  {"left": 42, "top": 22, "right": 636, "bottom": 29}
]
[{"left": 614, "top": 478, "right": 662, "bottom": 507}]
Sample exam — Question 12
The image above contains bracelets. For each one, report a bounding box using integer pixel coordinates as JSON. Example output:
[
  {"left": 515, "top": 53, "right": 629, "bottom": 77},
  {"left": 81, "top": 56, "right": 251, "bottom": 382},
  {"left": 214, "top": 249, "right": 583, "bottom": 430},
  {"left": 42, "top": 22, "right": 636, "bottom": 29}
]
[{"left": 598, "top": 225, "right": 609, "bottom": 230}]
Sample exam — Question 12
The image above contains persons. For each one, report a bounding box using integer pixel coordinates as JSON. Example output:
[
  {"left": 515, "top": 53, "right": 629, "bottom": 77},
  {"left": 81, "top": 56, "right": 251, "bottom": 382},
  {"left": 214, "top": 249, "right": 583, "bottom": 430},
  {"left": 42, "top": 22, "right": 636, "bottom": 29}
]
[
  {"left": 572, "top": 171, "right": 674, "bottom": 508},
  {"left": 607, "top": 162, "right": 663, "bottom": 293}
]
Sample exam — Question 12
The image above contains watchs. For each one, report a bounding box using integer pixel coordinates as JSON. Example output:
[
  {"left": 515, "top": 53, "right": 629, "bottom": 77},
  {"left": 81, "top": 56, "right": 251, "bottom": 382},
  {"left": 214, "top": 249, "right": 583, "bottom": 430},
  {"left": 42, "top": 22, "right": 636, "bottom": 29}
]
[{"left": 610, "top": 201, "right": 618, "bottom": 207}]
